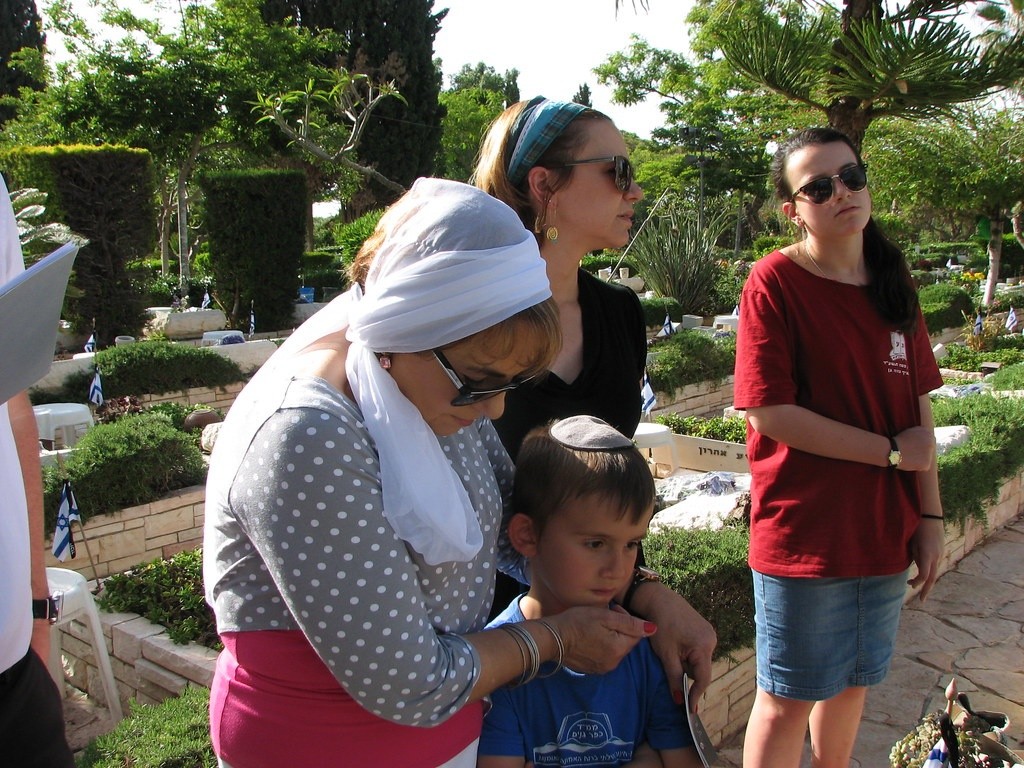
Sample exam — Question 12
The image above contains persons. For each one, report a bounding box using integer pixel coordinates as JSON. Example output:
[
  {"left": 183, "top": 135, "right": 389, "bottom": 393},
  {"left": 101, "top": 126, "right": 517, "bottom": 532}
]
[
  {"left": 475, "top": 414, "right": 705, "bottom": 768},
  {"left": 470, "top": 94, "right": 648, "bottom": 630},
  {"left": 734, "top": 129, "right": 946, "bottom": 768},
  {"left": 0, "top": 172, "right": 76, "bottom": 768},
  {"left": 203, "top": 176, "right": 717, "bottom": 768}
]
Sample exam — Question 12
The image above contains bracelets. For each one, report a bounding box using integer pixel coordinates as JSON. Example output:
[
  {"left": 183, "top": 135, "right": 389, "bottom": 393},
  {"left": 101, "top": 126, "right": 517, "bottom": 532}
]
[
  {"left": 920, "top": 514, "right": 944, "bottom": 520},
  {"left": 494, "top": 618, "right": 566, "bottom": 688}
]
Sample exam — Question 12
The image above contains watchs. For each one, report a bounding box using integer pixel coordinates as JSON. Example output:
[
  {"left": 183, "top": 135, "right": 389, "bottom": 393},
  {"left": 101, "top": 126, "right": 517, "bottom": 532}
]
[
  {"left": 32, "top": 590, "right": 64, "bottom": 625},
  {"left": 886, "top": 436, "right": 902, "bottom": 470},
  {"left": 622, "top": 565, "right": 661, "bottom": 609}
]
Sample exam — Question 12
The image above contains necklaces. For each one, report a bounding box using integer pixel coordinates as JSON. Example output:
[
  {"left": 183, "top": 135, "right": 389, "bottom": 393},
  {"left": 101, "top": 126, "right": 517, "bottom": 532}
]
[{"left": 805, "top": 241, "right": 830, "bottom": 280}]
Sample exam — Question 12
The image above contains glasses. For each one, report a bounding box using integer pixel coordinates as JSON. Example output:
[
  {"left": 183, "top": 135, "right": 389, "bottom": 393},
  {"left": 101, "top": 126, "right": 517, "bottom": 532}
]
[
  {"left": 543, "top": 155, "right": 634, "bottom": 193},
  {"left": 788, "top": 163, "right": 868, "bottom": 205},
  {"left": 428, "top": 346, "right": 540, "bottom": 406}
]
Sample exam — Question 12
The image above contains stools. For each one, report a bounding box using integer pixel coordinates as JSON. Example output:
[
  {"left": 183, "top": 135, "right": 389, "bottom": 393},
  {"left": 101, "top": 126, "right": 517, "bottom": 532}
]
[
  {"left": 203, "top": 331, "right": 244, "bottom": 346},
  {"left": 32, "top": 402, "right": 92, "bottom": 449},
  {"left": 713, "top": 315, "right": 738, "bottom": 331},
  {"left": 44, "top": 568, "right": 122, "bottom": 725},
  {"left": 633, "top": 422, "right": 679, "bottom": 475}
]
